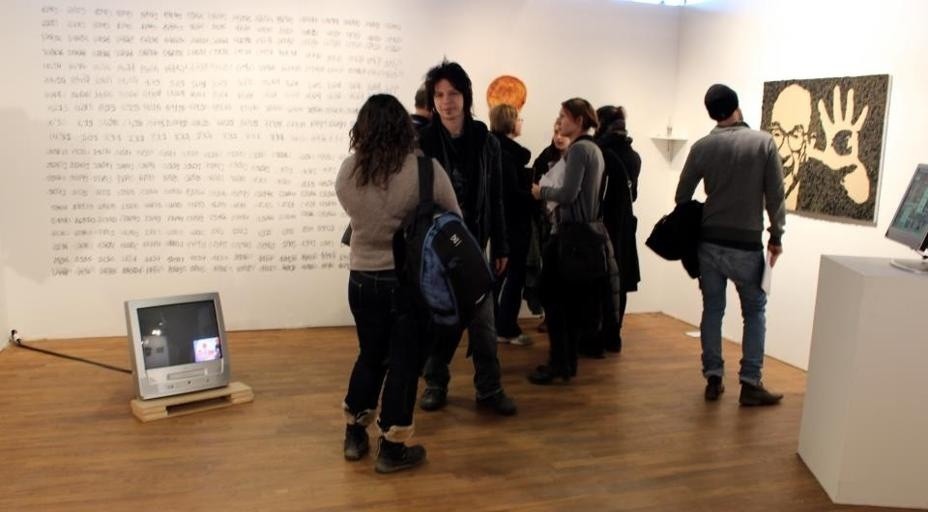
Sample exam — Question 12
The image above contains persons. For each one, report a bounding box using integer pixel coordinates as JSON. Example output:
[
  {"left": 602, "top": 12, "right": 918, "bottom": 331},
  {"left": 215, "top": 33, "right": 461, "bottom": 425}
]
[
  {"left": 675, "top": 83, "right": 785, "bottom": 405},
  {"left": 336, "top": 93, "right": 465, "bottom": 473},
  {"left": 767, "top": 82, "right": 873, "bottom": 213}
]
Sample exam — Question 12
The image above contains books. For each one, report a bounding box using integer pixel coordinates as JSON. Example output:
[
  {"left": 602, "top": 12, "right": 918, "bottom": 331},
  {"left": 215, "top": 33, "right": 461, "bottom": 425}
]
[{"left": 759, "top": 249, "right": 773, "bottom": 295}]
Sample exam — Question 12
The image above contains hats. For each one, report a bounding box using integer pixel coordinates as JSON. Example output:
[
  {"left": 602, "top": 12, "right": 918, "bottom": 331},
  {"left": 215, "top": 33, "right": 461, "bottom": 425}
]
[{"left": 704, "top": 84, "right": 739, "bottom": 118}]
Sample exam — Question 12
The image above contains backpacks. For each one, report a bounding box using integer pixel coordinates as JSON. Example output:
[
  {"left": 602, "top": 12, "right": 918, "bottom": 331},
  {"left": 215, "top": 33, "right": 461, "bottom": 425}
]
[{"left": 394, "top": 155, "right": 491, "bottom": 327}]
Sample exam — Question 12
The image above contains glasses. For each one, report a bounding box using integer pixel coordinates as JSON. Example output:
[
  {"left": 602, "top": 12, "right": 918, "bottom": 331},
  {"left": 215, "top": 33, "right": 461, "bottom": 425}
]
[{"left": 767, "top": 125, "right": 813, "bottom": 154}]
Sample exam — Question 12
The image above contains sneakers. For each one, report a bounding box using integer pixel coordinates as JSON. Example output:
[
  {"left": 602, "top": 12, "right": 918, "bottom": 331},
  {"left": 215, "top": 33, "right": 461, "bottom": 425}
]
[
  {"left": 528, "top": 365, "right": 572, "bottom": 384},
  {"left": 374, "top": 436, "right": 427, "bottom": 471},
  {"left": 738, "top": 381, "right": 785, "bottom": 407},
  {"left": 704, "top": 375, "right": 725, "bottom": 401},
  {"left": 343, "top": 422, "right": 370, "bottom": 460},
  {"left": 476, "top": 387, "right": 518, "bottom": 416},
  {"left": 420, "top": 378, "right": 448, "bottom": 411},
  {"left": 497, "top": 319, "right": 622, "bottom": 357}
]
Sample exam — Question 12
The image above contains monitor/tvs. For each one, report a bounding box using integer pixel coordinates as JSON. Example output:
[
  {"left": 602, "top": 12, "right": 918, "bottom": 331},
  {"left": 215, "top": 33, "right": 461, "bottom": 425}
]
[
  {"left": 123, "top": 291, "right": 230, "bottom": 400},
  {"left": 885, "top": 163, "right": 928, "bottom": 274}
]
